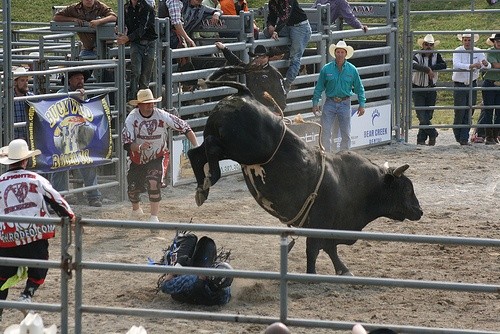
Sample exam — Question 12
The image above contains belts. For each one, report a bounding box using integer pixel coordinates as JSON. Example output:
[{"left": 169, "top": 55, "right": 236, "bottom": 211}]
[
  {"left": 295, "top": 20, "right": 309, "bottom": 26},
  {"left": 331, "top": 95, "right": 350, "bottom": 102}
]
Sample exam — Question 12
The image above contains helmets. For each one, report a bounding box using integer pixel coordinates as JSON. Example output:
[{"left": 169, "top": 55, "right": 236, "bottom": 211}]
[{"left": 211, "top": 263, "right": 235, "bottom": 289}]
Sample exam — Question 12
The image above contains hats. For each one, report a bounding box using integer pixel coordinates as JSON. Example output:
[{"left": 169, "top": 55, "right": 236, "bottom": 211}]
[
  {"left": 127, "top": 89, "right": 163, "bottom": 107},
  {"left": 114, "top": 325, "right": 149, "bottom": 334},
  {"left": 5, "top": 314, "right": 57, "bottom": 334},
  {"left": 58, "top": 66, "right": 93, "bottom": 83},
  {"left": 0, "top": 139, "right": 41, "bottom": 165},
  {"left": 458, "top": 29, "right": 479, "bottom": 42},
  {"left": 13, "top": 68, "right": 29, "bottom": 80},
  {"left": 486, "top": 34, "right": 500, "bottom": 46},
  {"left": 248, "top": 45, "right": 274, "bottom": 58},
  {"left": 418, "top": 34, "right": 441, "bottom": 47},
  {"left": 329, "top": 41, "right": 353, "bottom": 59}
]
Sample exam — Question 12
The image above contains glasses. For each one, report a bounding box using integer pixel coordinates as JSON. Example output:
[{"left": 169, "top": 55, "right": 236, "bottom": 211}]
[
  {"left": 496, "top": 40, "right": 500, "bottom": 43},
  {"left": 255, "top": 54, "right": 268, "bottom": 59},
  {"left": 463, "top": 38, "right": 470, "bottom": 42},
  {"left": 424, "top": 42, "right": 434, "bottom": 47}
]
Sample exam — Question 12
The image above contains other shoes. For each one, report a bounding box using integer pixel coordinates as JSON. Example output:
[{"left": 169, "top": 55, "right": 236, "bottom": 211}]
[
  {"left": 485, "top": 137, "right": 496, "bottom": 145},
  {"left": 284, "top": 80, "right": 291, "bottom": 93},
  {"left": 89, "top": 197, "right": 102, "bottom": 207},
  {"left": 471, "top": 132, "right": 484, "bottom": 143},
  {"left": 417, "top": 131, "right": 438, "bottom": 145},
  {"left": 129, "top": 208, "right": 143, "bottom": 220},
  {"left": 149, "top": 216, "right": 159, "bottom": 232},
  {"left": 456, "top": 139, "right": 468, "bottom": 145},
  {"left": 497, "top": 136, "right": 500, "bottom": 143}
]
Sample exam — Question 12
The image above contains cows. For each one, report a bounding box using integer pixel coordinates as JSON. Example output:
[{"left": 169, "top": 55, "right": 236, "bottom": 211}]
[{"left": 185, "top": 55, "right": 424, "bottom": 276}]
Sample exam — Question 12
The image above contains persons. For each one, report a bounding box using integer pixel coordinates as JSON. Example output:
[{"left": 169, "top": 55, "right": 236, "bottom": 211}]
[
  {"left": 2, "top": 67, "right": 42, "bottom": 151},
  {"left": 121, "top": 88, "right": 200, "bottom": 233},
  {"left": 147, "top": 232, "right": 234, "bottom": 307},
  {"left": 0, "top": 136, "right": 77, "bottom": 322},
  {"left": 450, "top": 28, "right": 492, "bottom": 145},
  {"left": 470, "top": 33, "right": 496, "bottom": 144},
  {"left": 310, "top": 0, "right": 368, "bottom": 34},
  {"left": 263, "top": 322, "right": 290, "bottom": 334},
  {"left": 56, "top": 70, "right": 93, "bottom": 95},
  {"left": 310, "top": 39, "right": 366, "bottom": 154},
  {"left": 261, "top": 0, "right": 312, "bottom": 92},
  {"left": 412, "top": 33, "right": 448, "bottom": 147},
  {"left": 52, "top": 0, "right": 118, "bottom": 87},
  {"left": 4, "top": 312, "right": 58, "bottom": 334},
  {"left": 214, "top": 41, "right": 288, "bottom": 116},
  {"left": 481, "top": 33, "right": 500, "bottom": 145},
  {"left": 158, "top": 0, "right": 260, "bottom": 91},
  {"left": 352, "top": 323, "right": 398, "bottom": 334},
  {"left": 114, "top": 0, "right": 158, "bottom": 102},
  {"left": 126, "top": 325, "right": 146, "bottom": 334}
]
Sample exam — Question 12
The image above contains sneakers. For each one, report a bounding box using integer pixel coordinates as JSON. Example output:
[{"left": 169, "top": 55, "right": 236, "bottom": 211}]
[{"left": 17, "top": 296, "right": 34, "bottom": 317}]
